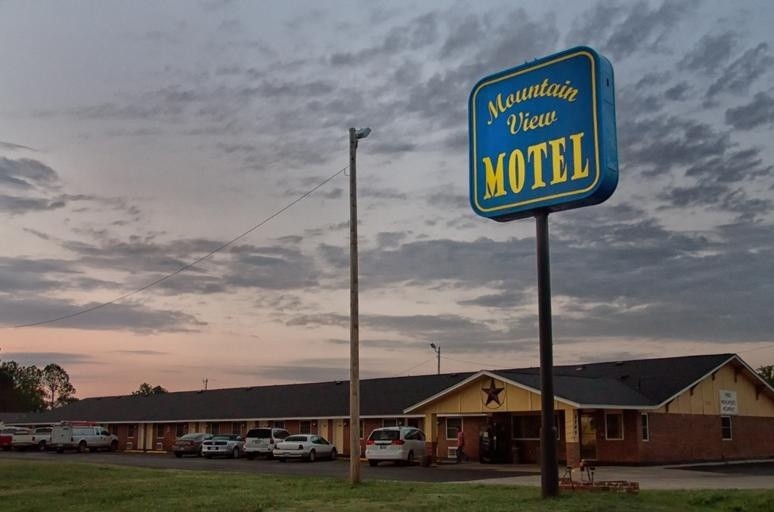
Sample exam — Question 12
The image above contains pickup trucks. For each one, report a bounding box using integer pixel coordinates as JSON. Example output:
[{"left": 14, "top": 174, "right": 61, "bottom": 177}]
[{"left": 0, "top": 420, "right": 120, "bottom": 453}]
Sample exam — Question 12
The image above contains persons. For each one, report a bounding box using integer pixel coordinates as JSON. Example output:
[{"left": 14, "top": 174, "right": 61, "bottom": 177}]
[{"left": 456, "top": 426, "right": 464, "bottom": 464}]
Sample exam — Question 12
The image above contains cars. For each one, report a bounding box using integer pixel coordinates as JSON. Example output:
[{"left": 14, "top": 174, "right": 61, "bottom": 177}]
[
  {"left": 170, "top": 428, "right": 337, "bottom": 463},
  {"left": 363, "top": 427, "right": 425, "bottom": 466}
]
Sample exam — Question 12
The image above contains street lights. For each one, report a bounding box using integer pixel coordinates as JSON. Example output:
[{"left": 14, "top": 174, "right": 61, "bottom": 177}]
[
  {"left": 348, "top": 124, "right": 372, "bottom": 485},
  {"left": 430, "top": 342, "right": 441, "bottom": 375}
]
